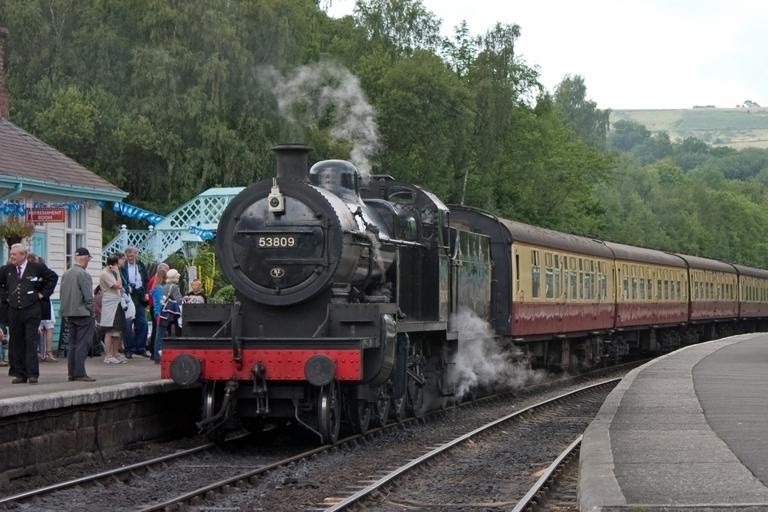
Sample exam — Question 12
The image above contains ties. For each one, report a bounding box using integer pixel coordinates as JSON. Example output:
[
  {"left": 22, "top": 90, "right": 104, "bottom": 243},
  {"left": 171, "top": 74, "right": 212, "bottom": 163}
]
[{"left": 17, "top": 266, "right": 21, "bottom": 277}]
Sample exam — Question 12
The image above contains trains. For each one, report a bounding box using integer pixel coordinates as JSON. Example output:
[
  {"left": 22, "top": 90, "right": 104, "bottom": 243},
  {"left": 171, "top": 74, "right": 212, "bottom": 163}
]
[{"left": 157, "top": 144, "right": 766, "bottom": 440}]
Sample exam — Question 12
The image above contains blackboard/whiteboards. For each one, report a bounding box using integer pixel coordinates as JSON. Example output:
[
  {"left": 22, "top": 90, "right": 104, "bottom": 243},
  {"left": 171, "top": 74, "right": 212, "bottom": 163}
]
[{"left": 59, "top": 315, "right": 70, "bottom": 350}]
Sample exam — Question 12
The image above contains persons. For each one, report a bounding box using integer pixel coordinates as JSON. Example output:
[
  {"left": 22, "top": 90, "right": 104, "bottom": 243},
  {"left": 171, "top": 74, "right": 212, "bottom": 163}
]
[
  {"left": 95, "top": 243, "right": 208, "bottom": 365},
  {"left": 60, "top": 247, "right": 96, "bottom": 383},
  {"left": 0, "top": 239, "right": 59, "bottom": 383}
]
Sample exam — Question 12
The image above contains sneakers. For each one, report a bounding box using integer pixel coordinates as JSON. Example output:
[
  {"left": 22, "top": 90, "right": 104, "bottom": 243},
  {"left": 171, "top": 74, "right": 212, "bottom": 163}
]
[{"left": 104, "top": 353, "right": 129, "bottom": 364}]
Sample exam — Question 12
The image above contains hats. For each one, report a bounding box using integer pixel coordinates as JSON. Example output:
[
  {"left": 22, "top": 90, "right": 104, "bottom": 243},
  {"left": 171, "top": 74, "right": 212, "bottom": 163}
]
[
  {"left": 76, "top": 248, "right": 92, "bottom": 258},
  {"left": 165, "top": 269, "right": 181, "bottom": 283}
]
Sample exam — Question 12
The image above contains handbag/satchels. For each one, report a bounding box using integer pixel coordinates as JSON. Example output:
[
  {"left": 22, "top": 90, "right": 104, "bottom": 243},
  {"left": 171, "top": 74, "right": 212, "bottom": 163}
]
[{"left": 160, "top": 295, "right": 181, "bottom": 322}]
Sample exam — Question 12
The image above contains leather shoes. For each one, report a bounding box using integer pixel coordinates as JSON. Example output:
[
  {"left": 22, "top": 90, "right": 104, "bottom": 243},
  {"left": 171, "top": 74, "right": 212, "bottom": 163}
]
[
  {"left": 126, "top": 351, "right": 133, "bottom": 358},
  {"left": 133, "top": 350, "right": 150, "bottom": 358},
  {"left": 12, "top": 378, "right": 27, "bottom": 383},
  {"left": 29, "top": 377, "right": 37, "bottom": 383},
  {"left": 69, "top": 374, "right": 96, "bottom": 381}
]
